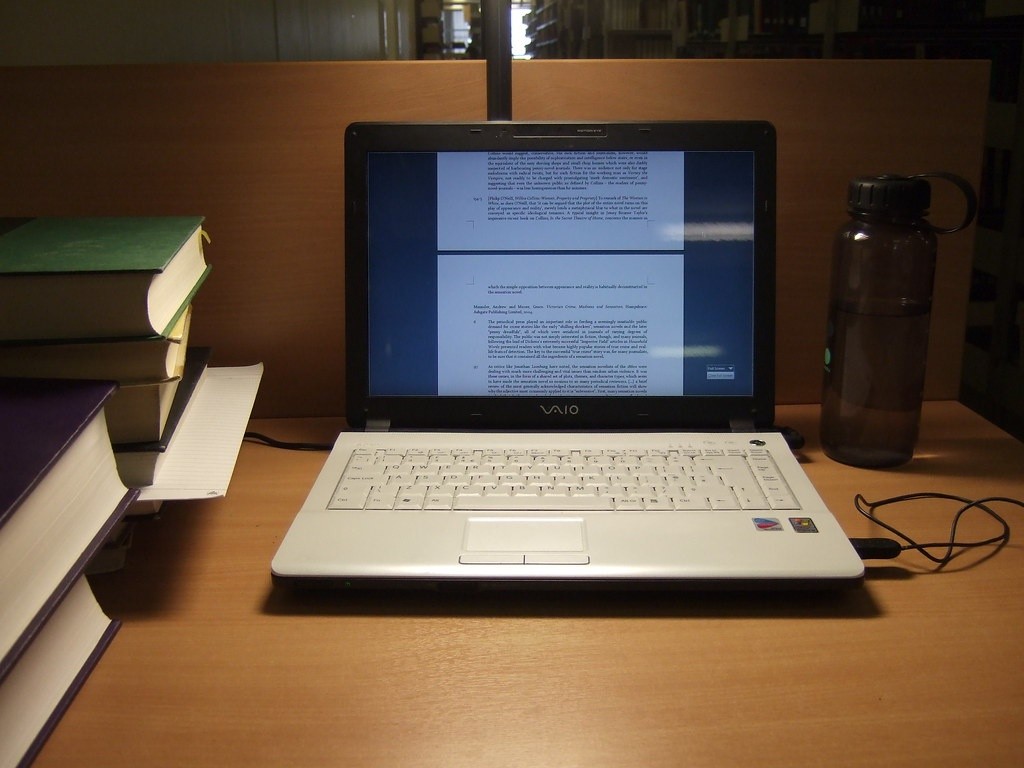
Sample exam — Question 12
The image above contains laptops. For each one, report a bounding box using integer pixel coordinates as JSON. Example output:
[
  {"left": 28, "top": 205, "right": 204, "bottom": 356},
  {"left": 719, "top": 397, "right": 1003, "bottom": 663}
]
[{"left": 269, "top": 118, "right": 867, "bottom": 593}]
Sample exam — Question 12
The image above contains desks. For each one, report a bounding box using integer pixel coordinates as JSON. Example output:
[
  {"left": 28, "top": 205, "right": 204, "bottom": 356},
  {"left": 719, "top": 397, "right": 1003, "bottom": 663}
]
[{"left": 0, "top": 59, "right": 1024, "bottom": 768}]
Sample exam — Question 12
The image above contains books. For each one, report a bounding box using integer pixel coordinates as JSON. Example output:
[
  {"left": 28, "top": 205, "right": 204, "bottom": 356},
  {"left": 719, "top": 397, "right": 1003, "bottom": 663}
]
[
  {"left": 523, "top": 0, "right": 1024, "bottom": 59},
  {"left": 0, "top": 215, "right": 214, "bottom": 768}
]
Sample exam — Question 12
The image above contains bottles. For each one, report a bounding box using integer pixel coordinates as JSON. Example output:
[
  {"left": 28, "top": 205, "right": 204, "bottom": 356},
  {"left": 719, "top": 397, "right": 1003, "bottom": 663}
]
[{"left": 820, "top": 173, "right": 977, "bottom": 467}]
